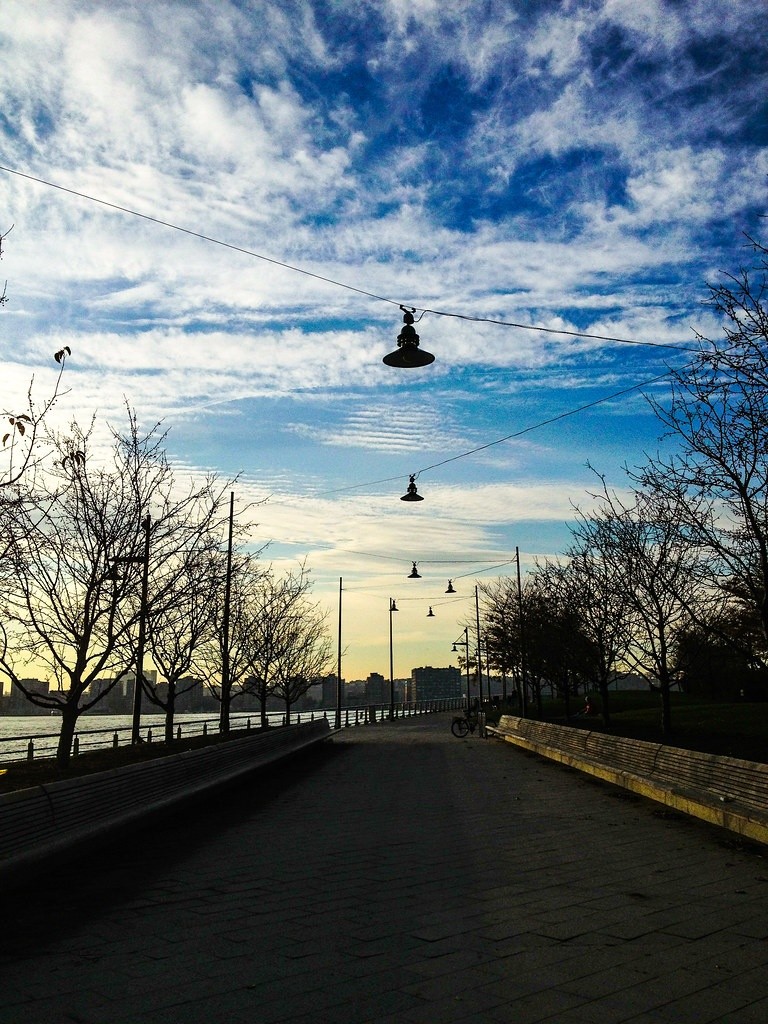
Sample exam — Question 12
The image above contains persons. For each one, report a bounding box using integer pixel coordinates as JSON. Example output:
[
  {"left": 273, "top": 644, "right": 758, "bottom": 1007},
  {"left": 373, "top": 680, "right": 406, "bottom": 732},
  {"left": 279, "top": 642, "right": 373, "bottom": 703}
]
[
  {"left": 470, "top": 697, "right": 479, "bottom": 711},
  {"left": 571, "top": 696, "right": 594, "bottom": 720},
  {"left": 512, "top": 688, "right": 517, "bottom": 695}
]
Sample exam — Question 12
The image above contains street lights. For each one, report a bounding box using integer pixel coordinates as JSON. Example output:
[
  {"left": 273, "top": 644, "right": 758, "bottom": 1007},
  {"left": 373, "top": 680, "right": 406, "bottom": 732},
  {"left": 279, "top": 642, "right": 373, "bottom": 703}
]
[
  {"left": 103, "top": 514, "right": 152, "bottom": 744},
  {"left": 451, "top": 626, "right": 470, "bottom": 712}
]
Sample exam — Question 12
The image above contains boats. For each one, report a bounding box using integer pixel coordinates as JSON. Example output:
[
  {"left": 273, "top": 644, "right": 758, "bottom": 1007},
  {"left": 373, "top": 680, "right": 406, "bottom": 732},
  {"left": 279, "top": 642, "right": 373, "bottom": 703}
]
[{"left": 51, "top": 709, "right": 63, "bottom": 716}]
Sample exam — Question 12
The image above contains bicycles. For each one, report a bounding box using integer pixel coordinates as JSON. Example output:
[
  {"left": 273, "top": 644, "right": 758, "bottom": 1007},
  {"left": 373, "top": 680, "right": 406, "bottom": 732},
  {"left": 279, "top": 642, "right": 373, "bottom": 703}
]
[{"left": 451, "top": 703, "right": 501, "bottom": 740}]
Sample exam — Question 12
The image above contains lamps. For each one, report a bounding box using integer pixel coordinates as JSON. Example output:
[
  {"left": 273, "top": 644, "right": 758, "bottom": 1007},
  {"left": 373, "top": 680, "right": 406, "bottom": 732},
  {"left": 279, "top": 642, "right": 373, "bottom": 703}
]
[
  {"left": 445, "top": 580, "right": 456, "bottom": 593},
  {"left": 383, "top": 304, "right": 436, "bottom": 368},
  {"left": 401, "top": 474, "right": 424, "bottom": 501},
  {"left": 427, "top": 607, "right": 435, "bottom": 617},
  {"left": 407, "top": 562, "right": 422, "bottom": 578},
  {"left": 389, "top": 600, "right": 399, "bottom": 611}
]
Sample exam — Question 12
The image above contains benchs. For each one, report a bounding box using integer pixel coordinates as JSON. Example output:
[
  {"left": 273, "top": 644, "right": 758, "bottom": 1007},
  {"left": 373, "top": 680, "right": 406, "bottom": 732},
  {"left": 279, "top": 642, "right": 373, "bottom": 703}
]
[
  {"left": 484, "top": 714, "right": 768, "bottom": 845},
  {"left": 0, "top": 719, "right": 343, "bottom": 893}
]
